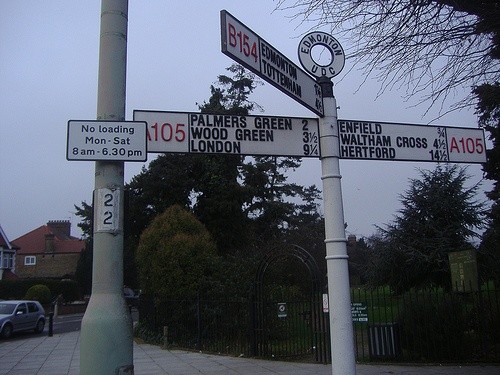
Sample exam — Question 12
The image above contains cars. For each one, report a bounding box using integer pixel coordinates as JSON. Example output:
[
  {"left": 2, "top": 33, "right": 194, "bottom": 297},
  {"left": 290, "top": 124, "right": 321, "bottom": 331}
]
[
  {"left": 123, "top": 288, "right": 139, "bottom": 305},
  {"left": 0, "top": 300, "right": 46, "bottom": 338}
]
